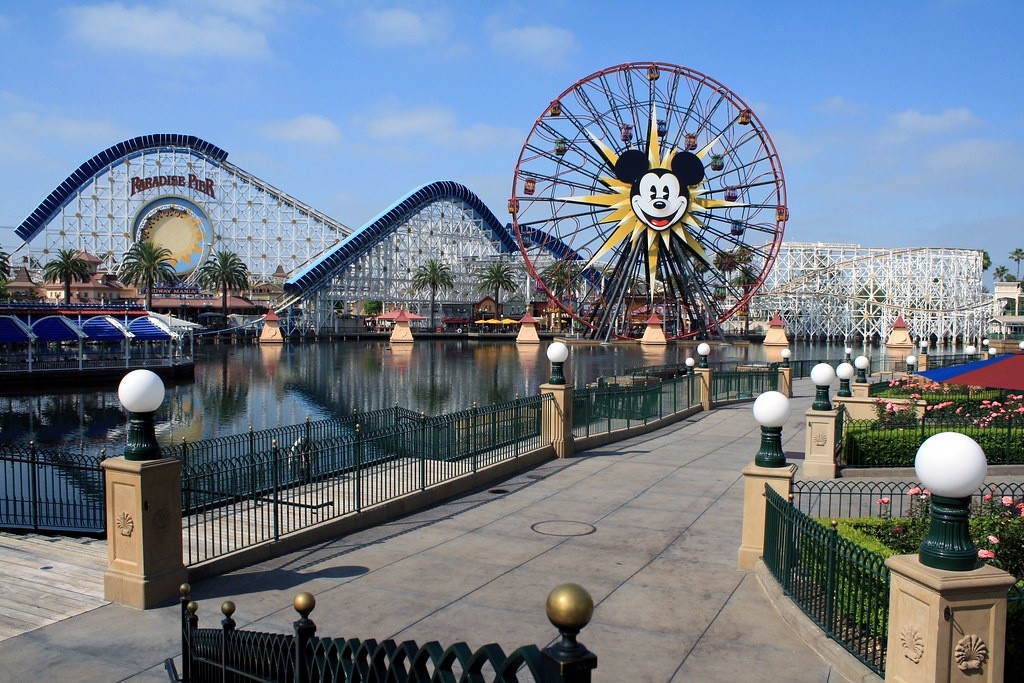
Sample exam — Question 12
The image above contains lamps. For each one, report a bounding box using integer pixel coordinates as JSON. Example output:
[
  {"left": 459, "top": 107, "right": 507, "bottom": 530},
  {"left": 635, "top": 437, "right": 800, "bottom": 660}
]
[
  {"left": 810, "top": 364, "right": 837, "bottom": 409},
  {"left": 854, "top": 356, "right": 869, "bottom": 382},
  {"left": 546, "top": 341, "right": 572, "bottom": 384},
  {"left": 967, "top": 344, "right": 976, "bottom": 360},
  {"left": 921, "top": 341, "right": 929, "bottom": 354},
  {"left": 845, "top": 346, "right": 853, "bottom": 360},
  {"left": 781, "top": 347, "right": 792, "bottom": 367},
  {"left": 836, "top": 362, "right": 856, "bottom": 396},
  {"left": 915, "top": 425, "right": 995, "bottom": 568},
  {"left": 685, "top": 357, "right": 695, "bottom": 374},
  {"left": 116, "top": 367, "right": 167, "bottom": 456},
  {"left": 748, "top": 390, "right": 794, "bottom": 463},
  {"left": 698, "top": 342, "right": 711, "bottom": 366},
  {"left": 906, "top": 356, "right": 917, "bottom": 373},
  {"left": 982, "top": 338, "right": 991, "bottom": 351},
  {"left": 1018, "top": 341, "right": 1024, "bottom": 351},
  {"left": 988, "top": 346, "right": 997, "bottom": 358}
]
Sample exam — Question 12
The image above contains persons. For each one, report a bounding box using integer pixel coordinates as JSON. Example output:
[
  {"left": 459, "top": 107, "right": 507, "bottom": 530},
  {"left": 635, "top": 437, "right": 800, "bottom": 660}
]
[{"left": 480, "top": 323, "right": 519, "bottom": 333}]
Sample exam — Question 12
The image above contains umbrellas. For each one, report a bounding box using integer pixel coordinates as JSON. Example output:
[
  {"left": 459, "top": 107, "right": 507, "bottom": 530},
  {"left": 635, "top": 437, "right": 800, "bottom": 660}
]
[
  {"left": 913, "top": 351, "right": 1024, "bottom": 390},
  {"left": 477, "top": 317, "right": 519, "bottom": 325}
]
